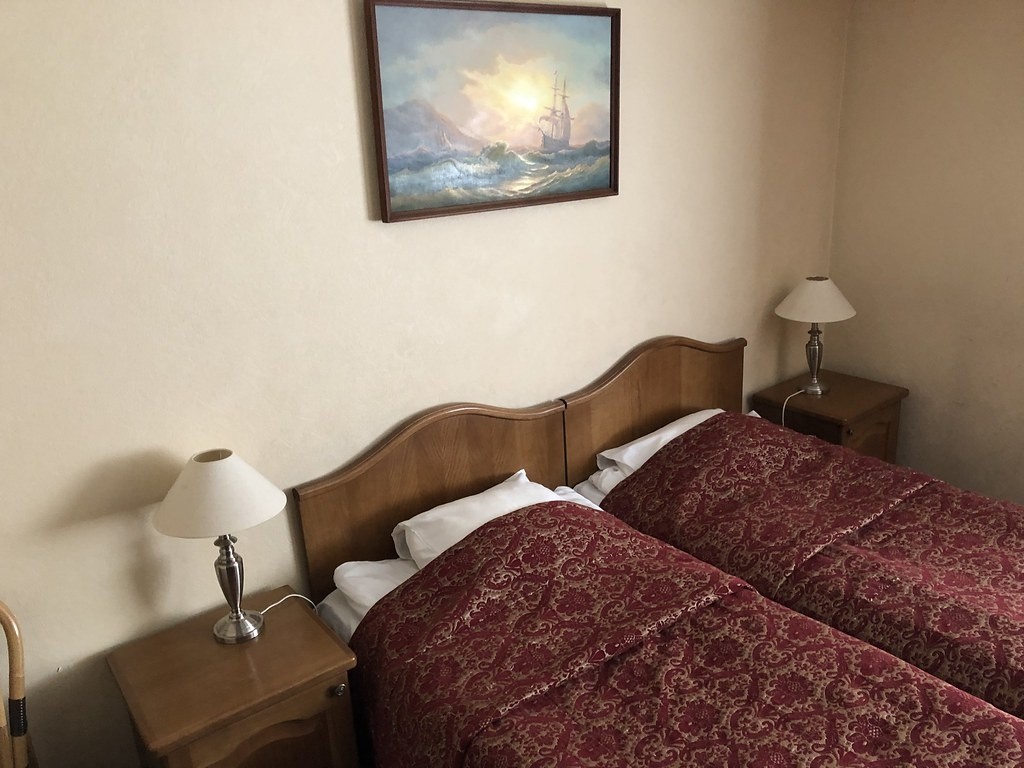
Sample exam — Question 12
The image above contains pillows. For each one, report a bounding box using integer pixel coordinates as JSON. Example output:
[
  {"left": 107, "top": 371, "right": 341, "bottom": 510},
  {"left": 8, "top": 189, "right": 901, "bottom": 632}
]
[
  {"left": 391, "top": 467, "right": 569, "bottom": 575},
  {"left": 593, "top": 406, "right": 726, "bottom": 482}
]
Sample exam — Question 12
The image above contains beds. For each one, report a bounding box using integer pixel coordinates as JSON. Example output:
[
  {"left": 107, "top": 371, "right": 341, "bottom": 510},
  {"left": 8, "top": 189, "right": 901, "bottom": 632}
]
[
  {"left": 291, "top": 399, "right": 1023, "bottom": 768},
  {"left": 562, "top": 332, "right": 1024, "bottom": 719}
]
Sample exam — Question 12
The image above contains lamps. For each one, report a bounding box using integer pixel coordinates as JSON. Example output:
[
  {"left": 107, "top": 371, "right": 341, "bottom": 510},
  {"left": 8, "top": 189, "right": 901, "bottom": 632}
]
[
  {"left": 151, "top": 446, "right": 289, "bottom": 646},
  {"left": 773, "top": 276, "right": 858, "bottom": 396}
]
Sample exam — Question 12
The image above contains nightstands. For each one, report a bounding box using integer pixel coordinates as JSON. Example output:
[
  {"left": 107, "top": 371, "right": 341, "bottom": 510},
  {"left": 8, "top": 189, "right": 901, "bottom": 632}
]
[
  {"left": 105, "top": 584, "right": 360, "bottom": 768},
  {"left": 750, "top": 366, "right": 910, "bottom": 467}
]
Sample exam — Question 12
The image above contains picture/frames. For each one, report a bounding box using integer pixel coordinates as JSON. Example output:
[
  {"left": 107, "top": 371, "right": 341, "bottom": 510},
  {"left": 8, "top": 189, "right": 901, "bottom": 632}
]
[{"left": 363, "top": 0, "right": 622, "bottom": 223}]
